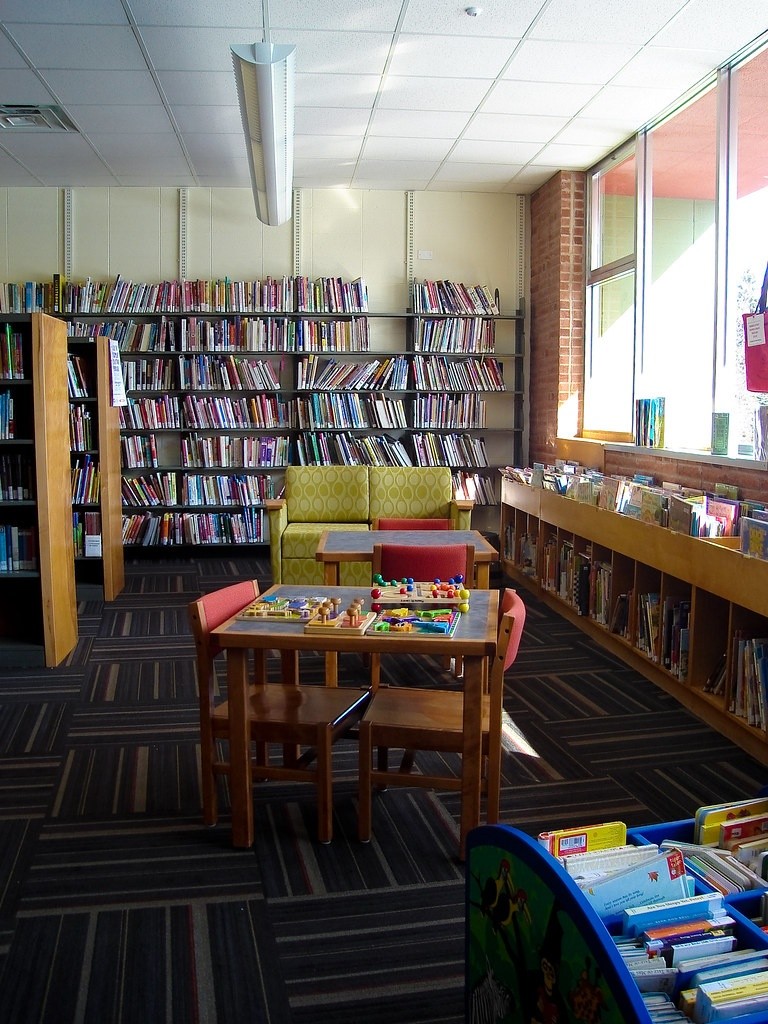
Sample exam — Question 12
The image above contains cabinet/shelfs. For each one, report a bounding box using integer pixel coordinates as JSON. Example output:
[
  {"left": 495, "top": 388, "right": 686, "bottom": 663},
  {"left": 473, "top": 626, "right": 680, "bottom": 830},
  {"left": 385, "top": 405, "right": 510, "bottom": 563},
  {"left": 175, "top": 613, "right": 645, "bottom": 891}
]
[
  {"left": 0, "top": 311, "right": 79, "bottom": 668},
  {"left": 500, "top": 473, "right": 768, "bottom": 768},
  {"left": 0, "top": 280, "right": 530, "bottom": 559},
  {"left": 67, "top": 336, "right": 125, "bottom": 601}
]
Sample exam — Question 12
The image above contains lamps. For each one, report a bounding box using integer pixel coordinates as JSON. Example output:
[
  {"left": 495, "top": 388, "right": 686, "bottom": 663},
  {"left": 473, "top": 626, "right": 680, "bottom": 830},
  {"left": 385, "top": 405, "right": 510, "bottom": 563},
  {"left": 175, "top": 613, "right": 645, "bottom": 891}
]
[{"left": 229, "top": 40, "right": 299, "bottom": 227}]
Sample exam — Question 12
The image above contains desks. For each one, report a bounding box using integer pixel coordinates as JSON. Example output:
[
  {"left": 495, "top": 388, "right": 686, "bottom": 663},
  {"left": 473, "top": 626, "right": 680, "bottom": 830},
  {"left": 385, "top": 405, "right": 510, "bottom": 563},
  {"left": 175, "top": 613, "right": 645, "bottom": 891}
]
[
  {"left": 315, "top": 530, "right": 500, "bottom": 589},
  {"left": 209, "top": 581, "right": 500, "bottom": 863}
]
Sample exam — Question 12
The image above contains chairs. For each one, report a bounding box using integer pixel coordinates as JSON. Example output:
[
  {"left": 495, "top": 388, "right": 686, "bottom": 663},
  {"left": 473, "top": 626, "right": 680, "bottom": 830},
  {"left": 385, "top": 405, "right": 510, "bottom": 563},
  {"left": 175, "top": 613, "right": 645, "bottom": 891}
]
[
  {"left": 356, "top": 588, "right": 527, "bottom": 843},
  {"left": 372, "top": 517, "right": 456, "bottom": 531},
  {"left": 187, "top": 579, "right": 372, "bottom": 846},
  {"left": 373, "top": 543, "right": 474, "bottom": 590}
]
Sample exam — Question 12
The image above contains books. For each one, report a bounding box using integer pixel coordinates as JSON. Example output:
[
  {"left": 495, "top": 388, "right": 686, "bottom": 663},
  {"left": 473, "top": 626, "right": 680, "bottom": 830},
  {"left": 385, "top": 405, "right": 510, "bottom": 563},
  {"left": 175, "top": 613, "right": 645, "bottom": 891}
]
[
  {"left": 535, "top": 797, "right": 768, "bottom": 1024},
  {"left": 1, "top": 270, "right": 768, "bottom": 731}
]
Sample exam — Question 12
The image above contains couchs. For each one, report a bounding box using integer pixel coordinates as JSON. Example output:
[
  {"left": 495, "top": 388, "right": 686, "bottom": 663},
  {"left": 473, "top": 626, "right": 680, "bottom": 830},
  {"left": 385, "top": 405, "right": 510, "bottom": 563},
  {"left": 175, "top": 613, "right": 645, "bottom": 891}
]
[{"left": 264, "top": 466, "right": 474, "bottom": 587}]
